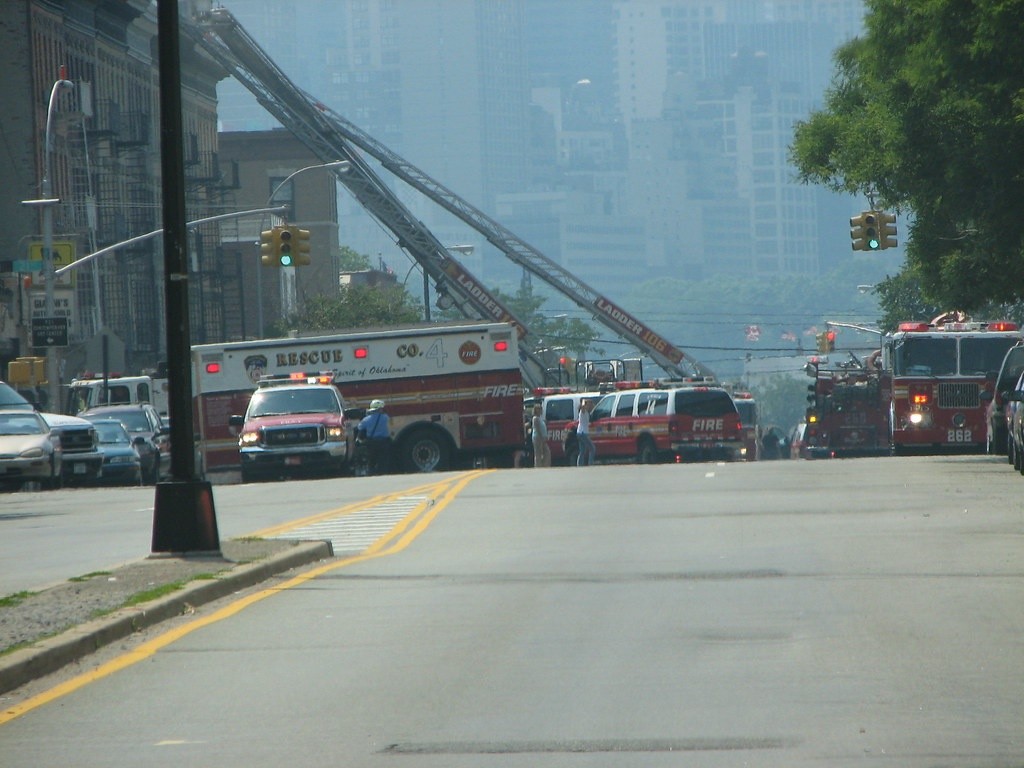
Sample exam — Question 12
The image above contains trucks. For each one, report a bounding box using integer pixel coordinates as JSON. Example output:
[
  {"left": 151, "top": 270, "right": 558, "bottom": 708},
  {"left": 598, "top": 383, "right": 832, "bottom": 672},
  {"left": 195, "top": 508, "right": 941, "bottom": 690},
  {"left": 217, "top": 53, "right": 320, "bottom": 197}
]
[{"left": 66, "top": 321, "right": 527, "bottom": 471}]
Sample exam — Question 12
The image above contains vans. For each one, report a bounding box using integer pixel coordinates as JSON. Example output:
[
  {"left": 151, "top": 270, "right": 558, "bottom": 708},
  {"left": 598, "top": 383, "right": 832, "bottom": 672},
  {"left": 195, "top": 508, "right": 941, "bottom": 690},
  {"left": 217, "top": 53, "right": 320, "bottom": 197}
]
[
  {"left": 731, "top": 392, "right": 757, "bottom": 461},
  {"left": 561, "top": 375, "right": 746, "bottom": 464},
  {"left": 533, "top": 386, "right": 617, "bottom": 466}
]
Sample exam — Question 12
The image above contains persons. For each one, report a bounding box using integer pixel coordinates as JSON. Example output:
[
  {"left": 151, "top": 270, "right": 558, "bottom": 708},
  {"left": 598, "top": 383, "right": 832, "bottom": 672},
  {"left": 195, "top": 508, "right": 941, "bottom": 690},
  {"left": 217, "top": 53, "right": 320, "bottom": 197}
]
[
  {"left": 576, "top": 399, "right": 596, "bottom": 466},
  {"left": 532, "top": 405, "right": 551, "bottom": 466},
  {"left": 562, "top": 427, "right": 577, "bottom": 465},
  {"left": 762, "top": 428, "right": 779, "bottom": 459},
  {"left": 358, "top": 400, "right": 389, "bottom": 474},
  {"left": 867, "top": 350, "right": 881, "bottom": 370}
]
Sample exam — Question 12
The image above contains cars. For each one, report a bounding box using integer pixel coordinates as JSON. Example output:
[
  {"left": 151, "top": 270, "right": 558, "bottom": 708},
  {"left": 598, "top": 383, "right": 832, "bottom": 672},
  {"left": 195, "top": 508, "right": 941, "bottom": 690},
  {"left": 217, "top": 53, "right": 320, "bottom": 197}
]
[
  {"left": 989, "top": 341, "right": 1024, "bottom": 477},
  {"left": 0, "top": 380, "right": 171, "bottom": 490}
]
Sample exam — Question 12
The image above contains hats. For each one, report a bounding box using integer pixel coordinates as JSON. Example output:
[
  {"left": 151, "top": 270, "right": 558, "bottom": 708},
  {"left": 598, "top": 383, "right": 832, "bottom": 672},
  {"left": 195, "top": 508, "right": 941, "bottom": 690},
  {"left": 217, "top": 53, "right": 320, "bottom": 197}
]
[{"left": 368, "top": 399, "right": 385, "bottom": 411}]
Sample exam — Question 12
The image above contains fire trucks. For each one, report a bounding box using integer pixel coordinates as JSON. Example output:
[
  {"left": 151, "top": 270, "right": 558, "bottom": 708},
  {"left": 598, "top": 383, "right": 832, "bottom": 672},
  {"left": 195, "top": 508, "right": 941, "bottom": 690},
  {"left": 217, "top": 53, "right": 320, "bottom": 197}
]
[
  {"left": 803, "top": 355, "right": 885, "bottom": 457},
  {"left": 879, "top": 320, "right": 1023, "bottom": 454}
]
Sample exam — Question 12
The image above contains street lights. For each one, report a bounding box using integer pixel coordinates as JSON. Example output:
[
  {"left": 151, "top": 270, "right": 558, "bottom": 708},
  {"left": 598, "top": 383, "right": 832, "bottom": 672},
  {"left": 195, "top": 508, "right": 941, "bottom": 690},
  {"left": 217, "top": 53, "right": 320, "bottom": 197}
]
[
  {"left": 42, "top": 78, "right": 76, "bottom": 415},
  {"left": 256, "top": 160, "right": 352, "bottom": 337},
  {"left": 423, "top": 244, "right": 474, "bottom": 321}
]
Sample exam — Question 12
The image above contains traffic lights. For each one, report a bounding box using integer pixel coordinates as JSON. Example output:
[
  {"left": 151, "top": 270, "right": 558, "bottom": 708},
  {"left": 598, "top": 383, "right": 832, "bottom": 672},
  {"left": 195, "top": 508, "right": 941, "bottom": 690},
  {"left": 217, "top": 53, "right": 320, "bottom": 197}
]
[
  {"left": 296, "top": 226, "right": 311, "bottom": 266},
  {"left": 260, "top": 229, "right": 275, "bottom": 267},
  {"left": 824, "top": 331, "right": 835, "bottom": 354},
  {"left": 275, "top": 224, "right": 297, "bottom": 266},
  {"left": 877, "top": 213, "right": 898, "bottom": 250},
  {"left": 861, "top": 211, "right": 881, "bottom": 251},
  {"left": 849, "top": 213, "right": 864, "bottom": 251},
  {"left": 816, "top": 333, "right": 826, "bottom": 353}
]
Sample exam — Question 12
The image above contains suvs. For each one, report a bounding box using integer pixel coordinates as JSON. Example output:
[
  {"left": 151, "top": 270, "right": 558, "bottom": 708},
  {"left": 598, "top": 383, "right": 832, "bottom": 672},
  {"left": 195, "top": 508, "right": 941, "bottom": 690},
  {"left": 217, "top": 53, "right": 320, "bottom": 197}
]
[{"left": 229, "top": 370, "right": 363, "bottom": 483}]
